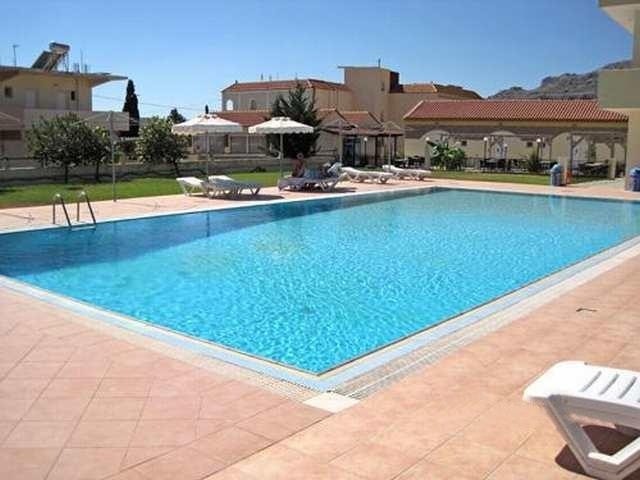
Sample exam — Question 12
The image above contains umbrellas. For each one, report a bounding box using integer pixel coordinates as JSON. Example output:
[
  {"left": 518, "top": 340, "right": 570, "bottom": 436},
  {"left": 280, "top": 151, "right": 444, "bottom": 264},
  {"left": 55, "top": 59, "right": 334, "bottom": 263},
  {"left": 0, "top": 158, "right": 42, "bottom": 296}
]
[
  {"left": 168, "top": 114, "right": 245, "bottom": 187},
  {"left": 248, "top": 113, "right": 311, "bottom": 178}
]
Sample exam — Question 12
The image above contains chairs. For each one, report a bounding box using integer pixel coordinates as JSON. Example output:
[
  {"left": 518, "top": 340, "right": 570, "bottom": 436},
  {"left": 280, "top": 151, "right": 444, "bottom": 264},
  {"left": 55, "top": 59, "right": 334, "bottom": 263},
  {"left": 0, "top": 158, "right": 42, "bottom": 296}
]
[
  {"left": 176, "top": 177, "right": 240, "bottom": 200},
  {"left": 208, "top": 175, "right": 262, "bottom": 196},
  {"left": 278, "top": 177, "right": 341, "bottom": 192},
  {"left": 340, "top": 167, "right": 393, "bottom": 184},
  {"left": 382, "top": 165, "right": 432, "bottom": 180},
  {"left": 523, "top": 360, "right": 640, "bottom": 480}
]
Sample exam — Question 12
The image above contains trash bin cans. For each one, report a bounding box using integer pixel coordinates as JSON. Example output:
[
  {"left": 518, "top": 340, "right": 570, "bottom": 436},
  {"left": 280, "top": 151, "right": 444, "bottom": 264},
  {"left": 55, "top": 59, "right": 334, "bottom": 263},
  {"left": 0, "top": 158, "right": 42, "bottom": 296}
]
[
  {"left": 630, "top": 167, "right": 640, "bottom": 192},
  {"left": 550, "top": 164, "right": 563, "bottom": 185}
]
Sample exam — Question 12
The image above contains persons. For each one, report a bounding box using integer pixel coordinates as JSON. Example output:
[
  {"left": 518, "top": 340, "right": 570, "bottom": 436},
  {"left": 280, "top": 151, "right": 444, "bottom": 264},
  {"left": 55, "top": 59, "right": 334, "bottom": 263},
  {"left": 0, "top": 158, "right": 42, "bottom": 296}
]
[{"left": 290, "top": 151, "right": 306, "bottom": 178}]
